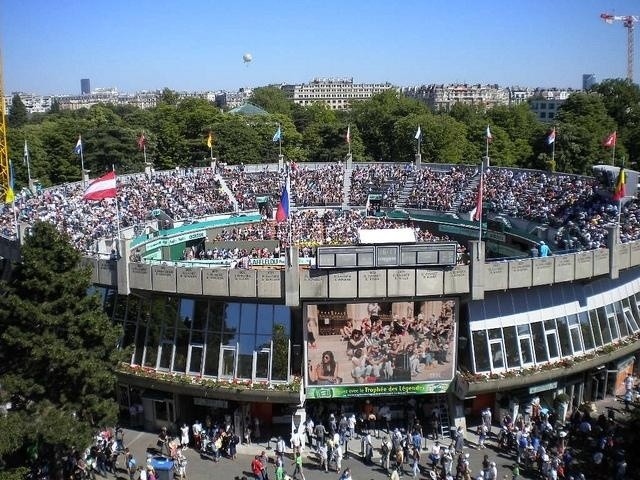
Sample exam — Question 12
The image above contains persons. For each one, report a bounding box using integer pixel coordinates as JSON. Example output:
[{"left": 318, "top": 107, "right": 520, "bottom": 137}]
[
  {"left": 307, "top": 302, "right": 456, "bottom": 385},
  {"left": 1, "top": 159, "right": 639, "bottom": 267},
  {"left": 70, "top": 370, "right": 640, "bottom": 480}
]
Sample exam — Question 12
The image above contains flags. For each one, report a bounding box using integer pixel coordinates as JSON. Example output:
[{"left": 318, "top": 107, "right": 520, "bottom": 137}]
[
  {"left": 544, "top": 125, "right": 556, "bottom": 147},
  {"left": 74, "top": 133, "right": 82, "bottom": 155},
  {"left": 346, "top": 123, "right": 351, "bottom": 145},
  {"left": 22, "top": 139, "right": 29, "bottom": 167},
  {"left": 273, "top": 124, "right": 281, "bottom": 143},
  {"left": 138, "top": 129, "right": 146, "bottom": 152},
  {"left": 8, "top": 159, "right": 15, "bottom": 190},
  {"left": 206, "top": 132, "right": 213, "bottom": 149},
  {"left": 601, "top": 130, "right": 616, "bottom": 150},
  {"left": 414, "top": 123, "right": 424, "bottom": 143},
  {"left": 485, "top": 124, "right": 493, "bottom": 146}
]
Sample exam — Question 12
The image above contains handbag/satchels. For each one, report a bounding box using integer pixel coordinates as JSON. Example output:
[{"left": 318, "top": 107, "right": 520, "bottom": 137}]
[
  {"left": 130, "top": 464, "right": 135, "bottom": 474},
  {"left": 428, "top": 452, "right": 435, "bottom": 461}
]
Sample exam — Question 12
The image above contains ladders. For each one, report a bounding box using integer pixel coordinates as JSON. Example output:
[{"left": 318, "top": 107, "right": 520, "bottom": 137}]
[{"left": 437, "top": 394, "right": 451, "bottom": 441}]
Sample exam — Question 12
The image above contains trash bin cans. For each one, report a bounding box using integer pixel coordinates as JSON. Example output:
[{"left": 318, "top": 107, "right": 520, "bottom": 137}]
[
  {"left": 151, "top": 456, "right": 175, "bottom": 480},
  {"left": 129, "top": 402, "right": 144, "bottom": 427}
]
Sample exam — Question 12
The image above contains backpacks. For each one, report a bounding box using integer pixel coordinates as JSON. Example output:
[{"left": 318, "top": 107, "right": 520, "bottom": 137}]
[
  {"left": 441, "top": 455, "right": 448, "bottom": 462},
  {"left": 233, "top": 436, "right": 239, "bottom": 444},
  {"left": 179, "top": 456, "right": 187, "bottom": 467},
  {"left": 457, "top": 461, "right": 466, "bottom": 472}
]
